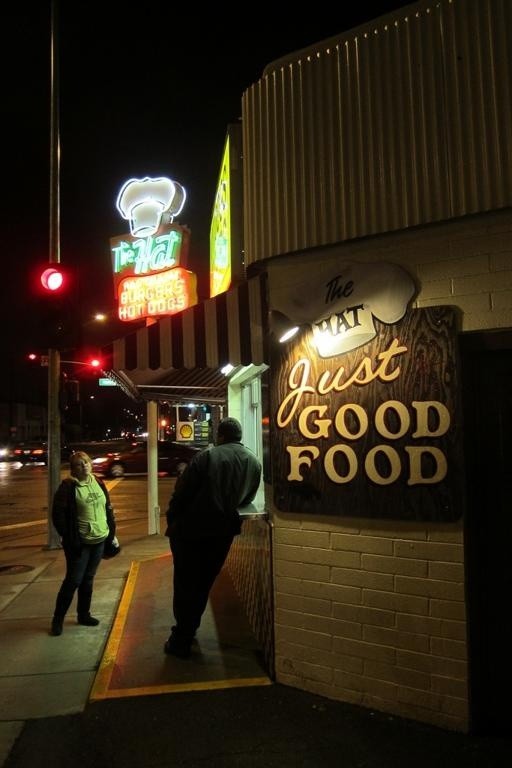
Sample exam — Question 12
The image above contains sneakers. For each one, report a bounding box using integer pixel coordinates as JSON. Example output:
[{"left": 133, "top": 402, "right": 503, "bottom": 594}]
[
  {"left": 162, "top": 624, "right": 201, "bottom": 658},
  {"left": 51, "top": 616, "right": 64, "bottom": 636},
  {"left": 77, "top": 611, "right": 99, "bottom": 626}
]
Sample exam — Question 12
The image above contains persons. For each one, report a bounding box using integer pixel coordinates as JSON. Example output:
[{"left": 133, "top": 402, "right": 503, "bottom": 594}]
[
  {"left": 165, "top": 418, "right": 261, "bottom": 659},
  {"left": 50, "top": 451, "right": 121, "bottom": 636}
]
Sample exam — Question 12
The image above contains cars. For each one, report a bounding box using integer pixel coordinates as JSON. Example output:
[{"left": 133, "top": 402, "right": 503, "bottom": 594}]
[
  {"left": 0, "top": 439, "right": 77, "bottom": 465},
  {"left": 92, "top": 440, "right": 201, "bottom": 478}
]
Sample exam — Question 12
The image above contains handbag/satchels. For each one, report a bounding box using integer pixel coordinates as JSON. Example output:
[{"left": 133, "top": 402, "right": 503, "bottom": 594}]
[{"left": 102, "top": 535, "right": 120, "bottom": 560}]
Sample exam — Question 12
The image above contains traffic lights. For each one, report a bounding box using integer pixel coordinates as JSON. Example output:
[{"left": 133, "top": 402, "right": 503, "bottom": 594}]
[{"left": 33, "top": 264, "right": 80, "bottom": 327}]
